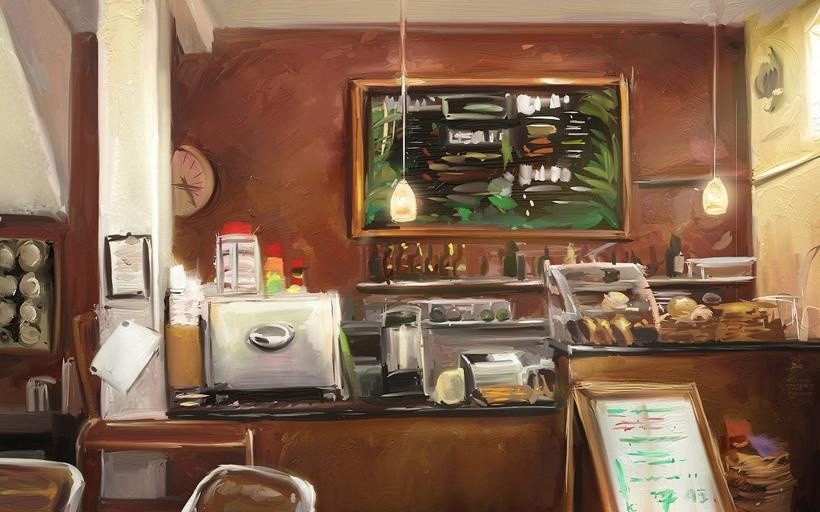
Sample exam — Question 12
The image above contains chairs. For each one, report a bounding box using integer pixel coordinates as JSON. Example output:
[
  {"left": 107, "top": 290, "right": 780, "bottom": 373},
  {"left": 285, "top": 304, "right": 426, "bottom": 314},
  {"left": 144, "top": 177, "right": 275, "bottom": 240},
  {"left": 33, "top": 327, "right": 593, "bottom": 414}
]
[
  {"left": 179, "top": 461, "right": 314, "bottom": 512},
  {"left": 0, "top": 456, "right": 88, "bottom": 511}
]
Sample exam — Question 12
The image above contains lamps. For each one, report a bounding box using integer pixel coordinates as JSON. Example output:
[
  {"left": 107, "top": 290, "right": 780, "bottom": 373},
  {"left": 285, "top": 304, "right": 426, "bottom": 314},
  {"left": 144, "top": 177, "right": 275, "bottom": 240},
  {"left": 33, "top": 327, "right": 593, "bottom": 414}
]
[
  {"left": 388, "top": 2, "right": 416, "bottom": 222},
  {"left": 702, "top": 8, "right": 728, "bottom": 219}
]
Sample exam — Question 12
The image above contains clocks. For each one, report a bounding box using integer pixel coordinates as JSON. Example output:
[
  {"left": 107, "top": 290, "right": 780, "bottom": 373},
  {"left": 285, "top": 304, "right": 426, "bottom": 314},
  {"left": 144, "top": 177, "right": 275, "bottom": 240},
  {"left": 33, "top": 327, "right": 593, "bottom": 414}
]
[{"left": 168, "top": 146, "right": 216, "bottom": 218}]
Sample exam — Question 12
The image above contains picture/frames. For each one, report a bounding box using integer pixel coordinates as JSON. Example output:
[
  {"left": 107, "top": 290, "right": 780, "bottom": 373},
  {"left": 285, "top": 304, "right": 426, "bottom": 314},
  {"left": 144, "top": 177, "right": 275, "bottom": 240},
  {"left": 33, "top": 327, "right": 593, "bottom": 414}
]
[
  {"left": 574, "top": 375, "right": 740, "bottom": 512},
  {"left": 349, "top": 75, "right": 631, "bottom": 244}
]
